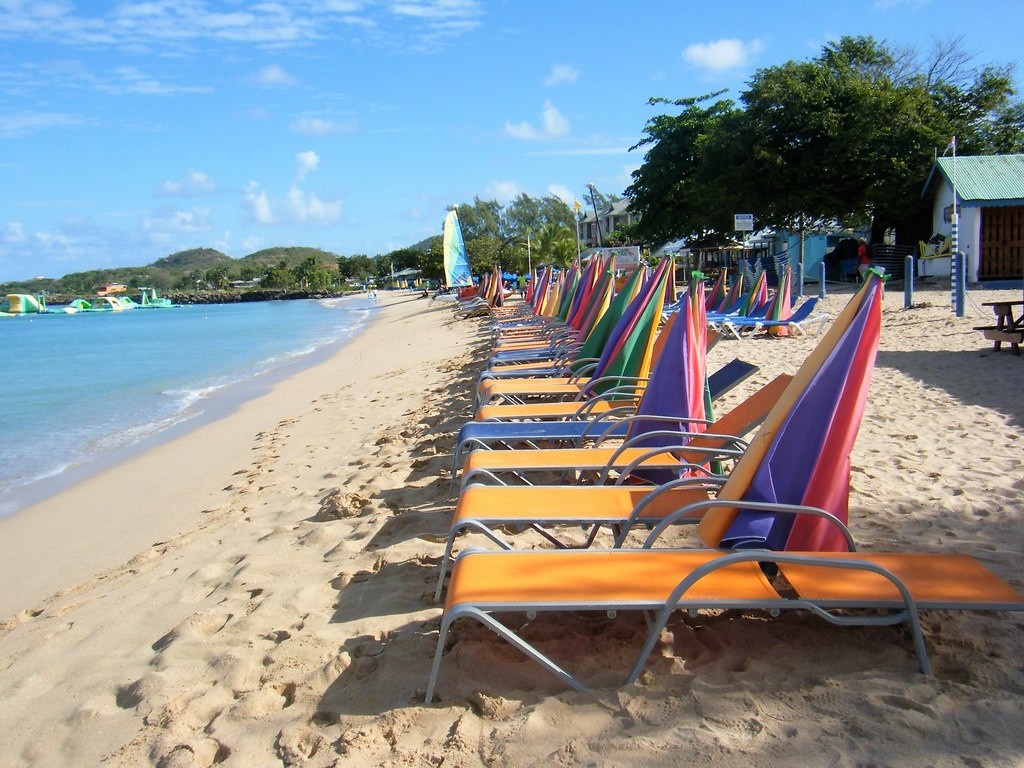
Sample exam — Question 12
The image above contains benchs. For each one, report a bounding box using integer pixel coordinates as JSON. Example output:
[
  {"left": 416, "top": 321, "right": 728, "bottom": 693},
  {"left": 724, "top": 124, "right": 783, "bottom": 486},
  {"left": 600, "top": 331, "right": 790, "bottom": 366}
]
[
  {"left": 1003, "top": 328, "right": 1024, "bottom": 335},
  {"left": 973, "top": 324, "right": 1024, "bottom": 330}
]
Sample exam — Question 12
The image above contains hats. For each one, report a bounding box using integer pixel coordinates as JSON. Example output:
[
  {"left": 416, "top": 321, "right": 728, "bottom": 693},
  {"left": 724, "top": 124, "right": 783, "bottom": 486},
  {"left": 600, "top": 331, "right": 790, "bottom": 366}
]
[{"left": 859, "top": 237, "right": 866, "bottom": 242}]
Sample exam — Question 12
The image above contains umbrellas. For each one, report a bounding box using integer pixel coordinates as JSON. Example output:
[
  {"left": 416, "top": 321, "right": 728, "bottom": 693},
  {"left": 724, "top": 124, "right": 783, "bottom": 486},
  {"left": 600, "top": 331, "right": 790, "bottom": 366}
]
[{"left": 475, "top": 248, "right": 888, "bottom": 558}]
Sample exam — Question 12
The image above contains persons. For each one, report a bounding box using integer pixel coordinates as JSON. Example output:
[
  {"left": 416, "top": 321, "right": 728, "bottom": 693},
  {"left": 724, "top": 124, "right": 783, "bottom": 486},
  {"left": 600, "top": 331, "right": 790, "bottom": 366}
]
[
  {"left": 517, "top": 272, "right": 527, "bottom": 299},
  {"left": 368, "top": 287, "right": 378, "bottom": 303}
]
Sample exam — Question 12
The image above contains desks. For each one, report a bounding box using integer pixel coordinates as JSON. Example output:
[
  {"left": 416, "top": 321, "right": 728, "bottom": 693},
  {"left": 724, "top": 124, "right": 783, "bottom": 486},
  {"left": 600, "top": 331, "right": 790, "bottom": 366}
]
[
  {"left": 919, "top": 237, "right": 953, "bottom": 259},
  {"left": 981, "top": 301, "right": 1024, "bottom": 355}
]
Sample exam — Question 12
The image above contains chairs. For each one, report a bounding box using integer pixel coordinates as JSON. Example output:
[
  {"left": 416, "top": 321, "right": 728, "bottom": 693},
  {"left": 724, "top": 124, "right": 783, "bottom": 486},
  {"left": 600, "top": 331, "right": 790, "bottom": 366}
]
[{"left": 425, "top": 249, "right": 1024, "bottom": 704}]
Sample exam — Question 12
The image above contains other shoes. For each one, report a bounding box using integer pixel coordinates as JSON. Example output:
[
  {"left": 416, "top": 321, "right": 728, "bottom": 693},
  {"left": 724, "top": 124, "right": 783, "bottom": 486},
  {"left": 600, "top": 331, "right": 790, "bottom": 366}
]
[{"left": 521, "top": 296, "right": 523, "bottom": 299}]
